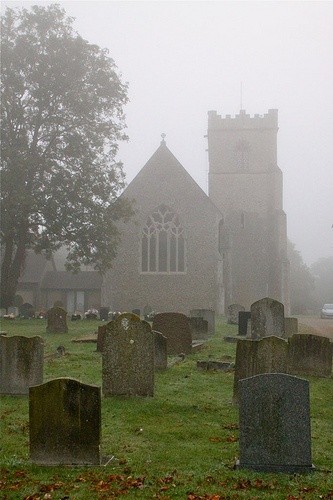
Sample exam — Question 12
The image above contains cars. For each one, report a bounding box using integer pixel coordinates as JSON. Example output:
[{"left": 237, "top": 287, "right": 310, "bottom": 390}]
[{"left": 319, "top": 303, "right": 333, "bottom": 318}]
